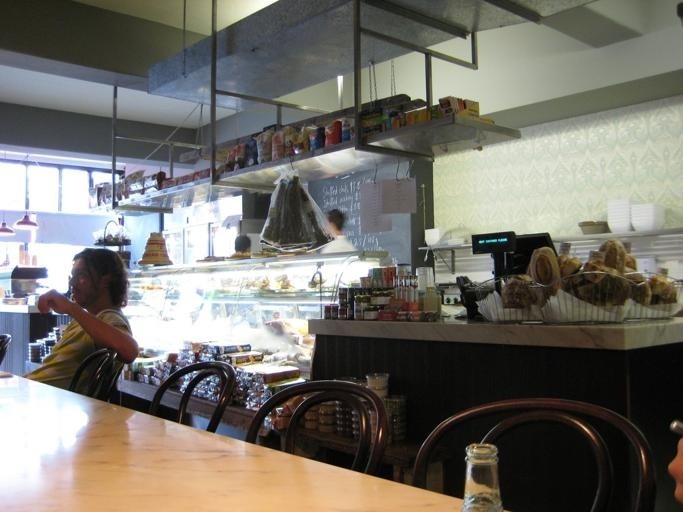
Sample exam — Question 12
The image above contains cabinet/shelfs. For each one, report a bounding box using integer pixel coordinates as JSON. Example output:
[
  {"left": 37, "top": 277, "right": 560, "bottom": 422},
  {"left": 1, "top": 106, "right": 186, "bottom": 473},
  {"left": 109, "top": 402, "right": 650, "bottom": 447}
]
[
  {"left": 463, "top": 442, "right": 504, "bottom": 512},
  {"left": 323, "top": 265, "right": 442, "bottom": 321}
]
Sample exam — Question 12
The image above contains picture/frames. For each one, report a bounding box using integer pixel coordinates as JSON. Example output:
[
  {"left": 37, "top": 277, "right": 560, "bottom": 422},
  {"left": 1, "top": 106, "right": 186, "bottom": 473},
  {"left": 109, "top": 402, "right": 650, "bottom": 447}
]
[{"left": 0, "top": 373, "right": 464, "bottom": 512}]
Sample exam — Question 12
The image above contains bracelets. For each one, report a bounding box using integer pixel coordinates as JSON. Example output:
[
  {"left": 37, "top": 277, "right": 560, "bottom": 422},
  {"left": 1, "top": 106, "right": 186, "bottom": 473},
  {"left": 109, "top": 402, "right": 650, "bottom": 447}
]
[{"left": 578, "top": 197, "right": 666, "bottom": 234}]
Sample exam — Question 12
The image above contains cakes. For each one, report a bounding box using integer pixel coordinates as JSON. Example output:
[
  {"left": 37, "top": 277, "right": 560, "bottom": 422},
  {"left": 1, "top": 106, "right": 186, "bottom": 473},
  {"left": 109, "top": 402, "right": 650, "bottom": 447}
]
[
  {"left": 418, "top": 290, "right": 441, "bottom": 317},
  {"left": 324, "top": 287, "right": 392, "bottom": 320}
]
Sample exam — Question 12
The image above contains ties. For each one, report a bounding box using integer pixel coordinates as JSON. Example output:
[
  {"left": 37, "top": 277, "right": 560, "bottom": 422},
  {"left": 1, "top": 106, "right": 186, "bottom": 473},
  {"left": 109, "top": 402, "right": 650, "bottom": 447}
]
[{"left": 492, "top": 233, "right": 557, "bottom": 282}]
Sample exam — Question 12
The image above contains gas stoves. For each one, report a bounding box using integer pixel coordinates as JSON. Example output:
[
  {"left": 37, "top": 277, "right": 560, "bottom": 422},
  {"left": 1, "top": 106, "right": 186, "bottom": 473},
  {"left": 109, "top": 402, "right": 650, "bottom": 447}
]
[{"left": 139, "top": 232, "right": 170, "bottom": 261}]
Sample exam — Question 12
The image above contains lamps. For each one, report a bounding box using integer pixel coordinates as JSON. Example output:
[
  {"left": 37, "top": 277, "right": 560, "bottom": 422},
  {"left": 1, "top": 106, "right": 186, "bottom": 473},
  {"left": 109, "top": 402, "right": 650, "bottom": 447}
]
[
  {"left": 245, "top": 380, "right": 388, "bottom": 476},
  {"left": 408, "top": 399, "right": 657, "bottom": 512},
  {"left": 149, "top": 362, "right": 236, "bottom": 433},
  {"left": 68, "top": 348, "right": 124, "bottom": 402},
  {"left": 0, "top": 333, "right": 12, "bottom": 366}
]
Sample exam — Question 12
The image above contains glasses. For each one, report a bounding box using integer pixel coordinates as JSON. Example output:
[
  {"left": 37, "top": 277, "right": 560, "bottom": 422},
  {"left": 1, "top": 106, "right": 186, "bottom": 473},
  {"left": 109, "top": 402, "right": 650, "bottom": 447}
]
[
  {"left": 0, "top": 152, "right": 13, "bottom": 233},
  {"left": 16, "top": 154, "right": 38, "bottom": 226}
]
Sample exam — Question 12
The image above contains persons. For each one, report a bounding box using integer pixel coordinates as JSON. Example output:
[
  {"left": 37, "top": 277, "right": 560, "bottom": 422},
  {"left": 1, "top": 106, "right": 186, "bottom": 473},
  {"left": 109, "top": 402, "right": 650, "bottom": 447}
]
[
  {"left": 234, "top": 235, "right": 251, "bottom": 253},
  {"left": 318, "top": 208, "right": 356, "bottom": 253},
  {"left": 22, "top": 248, "right": 138, "bottom": 389},
  {"left": 668, "top": 440, "right": 683, "bottom": 504}
]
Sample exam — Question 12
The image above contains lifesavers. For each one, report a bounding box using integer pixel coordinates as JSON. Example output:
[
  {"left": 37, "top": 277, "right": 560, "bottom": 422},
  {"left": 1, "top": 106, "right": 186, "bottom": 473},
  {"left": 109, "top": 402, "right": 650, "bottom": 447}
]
[{"left": 465, "top": 288, "right": 503, "bottom": 320}]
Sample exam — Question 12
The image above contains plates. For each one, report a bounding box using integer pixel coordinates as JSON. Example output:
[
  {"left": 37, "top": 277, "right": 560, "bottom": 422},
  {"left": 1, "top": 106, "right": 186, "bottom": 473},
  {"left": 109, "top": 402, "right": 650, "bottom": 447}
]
[{"left": 121, "top": 251, "right": 394, "bottom": 380}]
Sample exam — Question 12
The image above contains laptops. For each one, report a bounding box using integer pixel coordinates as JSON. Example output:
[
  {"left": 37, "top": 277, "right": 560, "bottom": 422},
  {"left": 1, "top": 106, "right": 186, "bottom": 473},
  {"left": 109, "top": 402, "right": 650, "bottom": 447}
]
[{"left": 499, "top": 238, "right": 678, "bottom": 307}]
